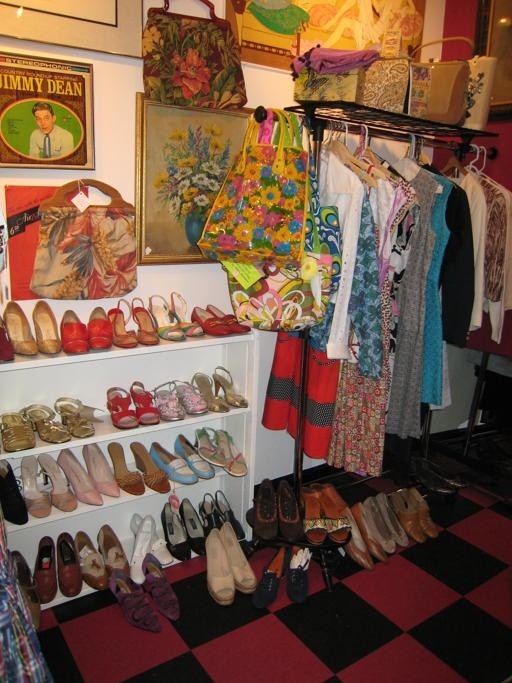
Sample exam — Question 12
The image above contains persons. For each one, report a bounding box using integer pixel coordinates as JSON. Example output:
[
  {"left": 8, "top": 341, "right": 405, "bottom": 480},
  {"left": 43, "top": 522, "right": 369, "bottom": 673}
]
[{"left": 29, "top": 101, "right": 75, "bottom": 160}]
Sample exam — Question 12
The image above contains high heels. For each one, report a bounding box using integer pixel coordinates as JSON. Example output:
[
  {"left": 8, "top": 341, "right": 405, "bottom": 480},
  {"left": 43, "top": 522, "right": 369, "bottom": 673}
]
[
  {"left": 1, "top": 426, "right": 248, "bottom": 525},
  {"left": 1, "top": 366, "right": 248, "bottom": 452},
  {"left": 1, "top": 293, "right": 250, "bottom": 362}
]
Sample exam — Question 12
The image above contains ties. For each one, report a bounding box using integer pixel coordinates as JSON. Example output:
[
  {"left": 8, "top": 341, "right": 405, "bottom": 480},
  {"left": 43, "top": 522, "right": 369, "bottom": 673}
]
[{"left": 44, "top": 134, "right": 51, "bottom": 158}]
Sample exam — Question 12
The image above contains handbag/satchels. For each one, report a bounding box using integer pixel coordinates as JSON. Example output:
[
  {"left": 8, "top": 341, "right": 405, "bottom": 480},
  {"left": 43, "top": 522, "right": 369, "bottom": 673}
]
[
  {"left": 357, "top": 56, "right": 468, "bottom": 125},
  {"left": 198, "top": 148, "right": 340, "bottom": 332},
  {"left": 142, "top": 9, "right": 248, "bottom": 110},
  {"left": 30, "top": 200, "right": 139, "bottom": 301}
]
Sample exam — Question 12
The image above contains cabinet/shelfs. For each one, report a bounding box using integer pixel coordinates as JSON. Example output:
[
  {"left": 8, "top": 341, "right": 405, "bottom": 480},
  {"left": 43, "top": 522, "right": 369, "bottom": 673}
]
[{"left": 0, "top": 325, "right": 261, "bottom": 613}]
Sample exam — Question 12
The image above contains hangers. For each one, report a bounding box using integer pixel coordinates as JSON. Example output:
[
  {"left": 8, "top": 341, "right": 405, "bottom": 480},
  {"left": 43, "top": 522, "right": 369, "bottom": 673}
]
[{"left": 320, "top": 118, "right": 512, "bottom": 199}]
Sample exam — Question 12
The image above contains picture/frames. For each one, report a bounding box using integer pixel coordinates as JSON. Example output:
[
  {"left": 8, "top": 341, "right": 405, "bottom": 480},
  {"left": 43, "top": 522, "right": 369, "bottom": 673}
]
[
  {"left": 474, "top": 0, "right": 512, "bottom": 119},
  {"left": 137, "top": 93, "right": 255, "bottom": 265},
  {"left": 1, "top": 51, "right": 95, "bottom": 170}
]
